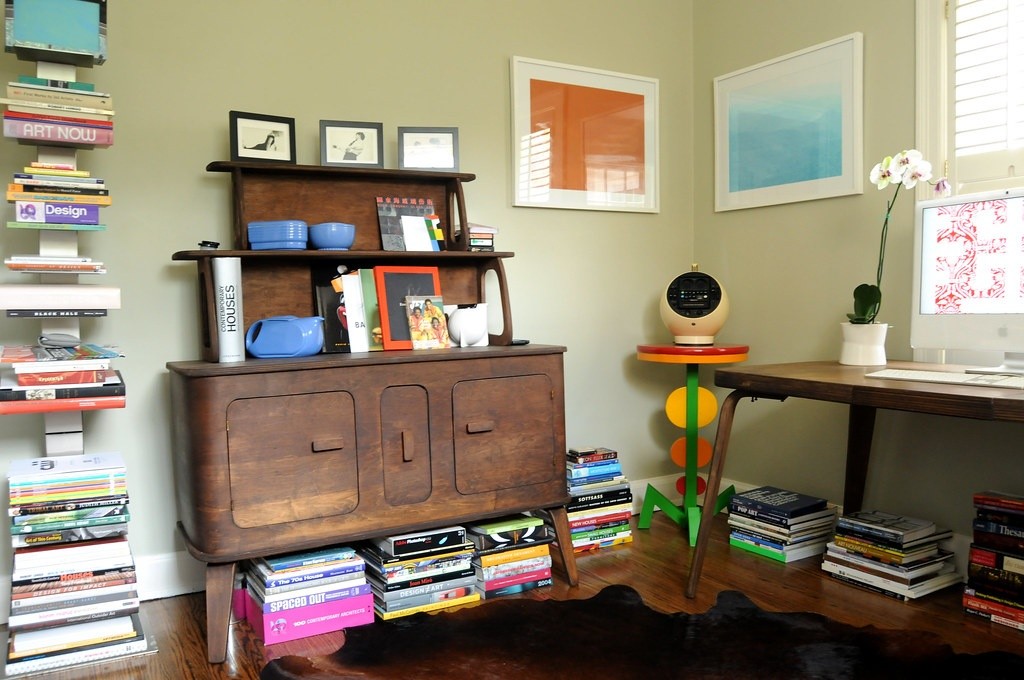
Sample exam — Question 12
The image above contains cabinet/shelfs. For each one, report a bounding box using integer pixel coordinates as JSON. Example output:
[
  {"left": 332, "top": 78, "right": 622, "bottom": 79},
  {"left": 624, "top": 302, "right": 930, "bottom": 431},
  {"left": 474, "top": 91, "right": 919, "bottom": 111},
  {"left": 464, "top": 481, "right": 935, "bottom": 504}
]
[
  {"left": 166, "top": 160, "right": 579, "bottom": 663},
  {"left": 1, "top": 1, "right": 159, "bottom": 680}
]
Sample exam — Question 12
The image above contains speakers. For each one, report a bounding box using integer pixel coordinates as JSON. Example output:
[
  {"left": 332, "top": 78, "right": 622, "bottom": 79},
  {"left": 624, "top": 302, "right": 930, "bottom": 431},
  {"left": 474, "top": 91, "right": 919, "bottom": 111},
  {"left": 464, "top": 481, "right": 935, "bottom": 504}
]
[{"left": 660, "top": 264, "right": 730, "bottom": 345}]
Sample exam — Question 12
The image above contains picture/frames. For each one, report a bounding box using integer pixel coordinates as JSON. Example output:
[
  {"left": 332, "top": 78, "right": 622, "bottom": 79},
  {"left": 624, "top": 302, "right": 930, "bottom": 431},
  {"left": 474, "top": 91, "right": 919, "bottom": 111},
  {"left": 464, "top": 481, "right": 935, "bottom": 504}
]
[
  {"left": 229, "top": 110, "right": 297, "bottom": 166},
  {"left": 510, "top": 54, "right": 661, "bottom": 214},
  {"left": 397, "top": 126, "right": 460, "bottom": 173},
  {"left": 319, "top": 119, "right": 384, "bottom": 170},
  {"left": 712, "top": 31, "right": 865, "bottom": 213}
]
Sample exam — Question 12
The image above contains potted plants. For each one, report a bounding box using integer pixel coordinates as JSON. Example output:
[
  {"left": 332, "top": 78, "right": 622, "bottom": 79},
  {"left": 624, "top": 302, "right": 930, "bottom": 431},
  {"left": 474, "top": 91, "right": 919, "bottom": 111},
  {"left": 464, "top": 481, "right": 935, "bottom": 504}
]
[{"left": 839, "top": 283, "right": 888, "bottom": 367}]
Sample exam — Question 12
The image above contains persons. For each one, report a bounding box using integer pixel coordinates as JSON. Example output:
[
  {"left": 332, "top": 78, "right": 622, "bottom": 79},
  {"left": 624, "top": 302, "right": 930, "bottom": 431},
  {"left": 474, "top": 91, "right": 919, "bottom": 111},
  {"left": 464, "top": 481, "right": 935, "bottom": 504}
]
[
  {"left": 243, "top": 130, "right": 283, "bottom": 151},
  {"left": 332, "top": 132, "right": 365, "bottom": 161},
  {"left": 409, "top": 298, "right": 449, "bottom": 349}
]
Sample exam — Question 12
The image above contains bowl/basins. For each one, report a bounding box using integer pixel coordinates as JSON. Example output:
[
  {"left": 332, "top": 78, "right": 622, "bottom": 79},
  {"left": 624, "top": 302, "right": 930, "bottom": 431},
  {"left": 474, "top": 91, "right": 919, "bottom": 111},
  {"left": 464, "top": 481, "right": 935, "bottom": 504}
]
[{"left": 309, "top": 221, "right": 356, "bottom": 251}]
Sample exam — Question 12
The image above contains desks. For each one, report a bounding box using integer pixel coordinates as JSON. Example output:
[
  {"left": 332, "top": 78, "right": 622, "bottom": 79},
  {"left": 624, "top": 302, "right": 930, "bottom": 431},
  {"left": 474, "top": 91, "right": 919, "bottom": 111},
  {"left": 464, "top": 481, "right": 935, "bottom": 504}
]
[{"left": 684, "top": 356, "right": 1024, "bottom": 599}]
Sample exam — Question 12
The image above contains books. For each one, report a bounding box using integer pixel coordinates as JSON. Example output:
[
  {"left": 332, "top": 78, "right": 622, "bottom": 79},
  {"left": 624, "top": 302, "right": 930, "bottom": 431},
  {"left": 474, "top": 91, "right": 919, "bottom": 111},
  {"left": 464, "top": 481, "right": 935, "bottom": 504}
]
[
  {"left": 0, "top": 74, "right": 146, "bottom": 677},
  {"left": 728, "top": 484, "right": 1023, "bottom": 632},
  {"left": 233, "top": 448, "right": 634, "bottom": 645}
]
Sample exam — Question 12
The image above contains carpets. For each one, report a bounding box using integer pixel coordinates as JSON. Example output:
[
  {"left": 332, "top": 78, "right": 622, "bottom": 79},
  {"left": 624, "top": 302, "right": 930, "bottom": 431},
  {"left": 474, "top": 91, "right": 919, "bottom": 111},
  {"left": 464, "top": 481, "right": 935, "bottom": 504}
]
[{"left": 257, "top": 582, "right": 1024, "bottom": 679}]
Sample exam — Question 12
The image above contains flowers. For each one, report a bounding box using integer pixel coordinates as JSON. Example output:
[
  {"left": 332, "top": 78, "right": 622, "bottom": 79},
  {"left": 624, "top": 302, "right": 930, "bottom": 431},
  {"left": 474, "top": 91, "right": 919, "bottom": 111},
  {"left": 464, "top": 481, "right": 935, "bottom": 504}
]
[{"left": 868, "top": 148, "right": 952, "bottom": 288}]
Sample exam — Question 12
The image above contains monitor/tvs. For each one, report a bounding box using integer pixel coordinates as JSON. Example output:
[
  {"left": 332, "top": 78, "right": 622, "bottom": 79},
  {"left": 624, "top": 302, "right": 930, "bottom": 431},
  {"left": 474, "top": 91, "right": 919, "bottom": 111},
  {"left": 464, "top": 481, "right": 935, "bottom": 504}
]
[{"left": 910, "top": 187, "right": 1023, "bottom": 375}]
